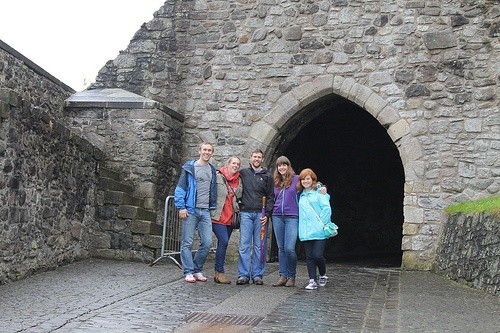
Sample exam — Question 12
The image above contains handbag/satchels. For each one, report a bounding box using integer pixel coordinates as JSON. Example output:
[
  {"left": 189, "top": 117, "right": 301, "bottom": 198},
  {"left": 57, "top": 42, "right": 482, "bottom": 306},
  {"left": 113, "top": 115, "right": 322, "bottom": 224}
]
[
  {"left": 323, "top": 222, "right": 338, "bottom": 238},
  {"left": 231, "top": 209, "right": 241, "bottom": 229}
]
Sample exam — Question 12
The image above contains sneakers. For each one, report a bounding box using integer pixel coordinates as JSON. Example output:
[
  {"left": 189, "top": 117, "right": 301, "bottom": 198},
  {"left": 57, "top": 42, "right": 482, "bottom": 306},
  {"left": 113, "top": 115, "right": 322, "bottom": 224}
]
[
  {"left": 304, "top": 278, "right": 318, "bottom": 290},
  {"left": 319, "top": 273, "right": 328, "bottom": 287},
  {"left": 183, "top": 273, "right": 197, "bottom": 283},
  {"left": 194, "top": 272, "right": 208, "bottom": 282}
]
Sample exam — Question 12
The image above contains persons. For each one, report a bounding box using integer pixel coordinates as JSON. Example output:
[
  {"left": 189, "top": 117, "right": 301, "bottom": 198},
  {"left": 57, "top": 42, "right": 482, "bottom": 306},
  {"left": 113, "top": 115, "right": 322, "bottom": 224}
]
[
  {"left": 271, "top": 156, "right": 328, "bottom": 287},
  {"left": 174, "top": 142, "right": 217, "bottom": 282},
  {"left": 260, "top": 196, "right": 266, "bottom": 268},
  {"left": 295, "top": 169, "right": 332, "bottom": 289},
  {"left": 215, "top": 149, "right": 274, "bottom": 285},
  {"left": 210, "top": 156, "right": 243, "bottom": 284}
]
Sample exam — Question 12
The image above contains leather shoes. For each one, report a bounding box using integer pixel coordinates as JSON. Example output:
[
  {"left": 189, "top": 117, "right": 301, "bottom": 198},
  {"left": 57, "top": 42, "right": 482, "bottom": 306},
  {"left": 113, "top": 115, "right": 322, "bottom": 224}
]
[
  {"left": 285, "top": 276, "right": 296, "bottom": 287},
  {"left": 272, "top": 274, "right": 287, "bottom": 286}
]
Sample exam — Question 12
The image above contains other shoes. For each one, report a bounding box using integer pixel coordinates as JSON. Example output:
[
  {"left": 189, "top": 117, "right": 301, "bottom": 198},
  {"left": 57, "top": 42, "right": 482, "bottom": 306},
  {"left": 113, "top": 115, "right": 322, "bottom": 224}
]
[
  {"left": 236, "top": 276, "right": 249, "bottom": 285},
  {"left": 253, "top": 275, "right": 264, "bottom": 285},
  {"left": 213, "top": 271, "right": 230, "bottom": 285}
]
